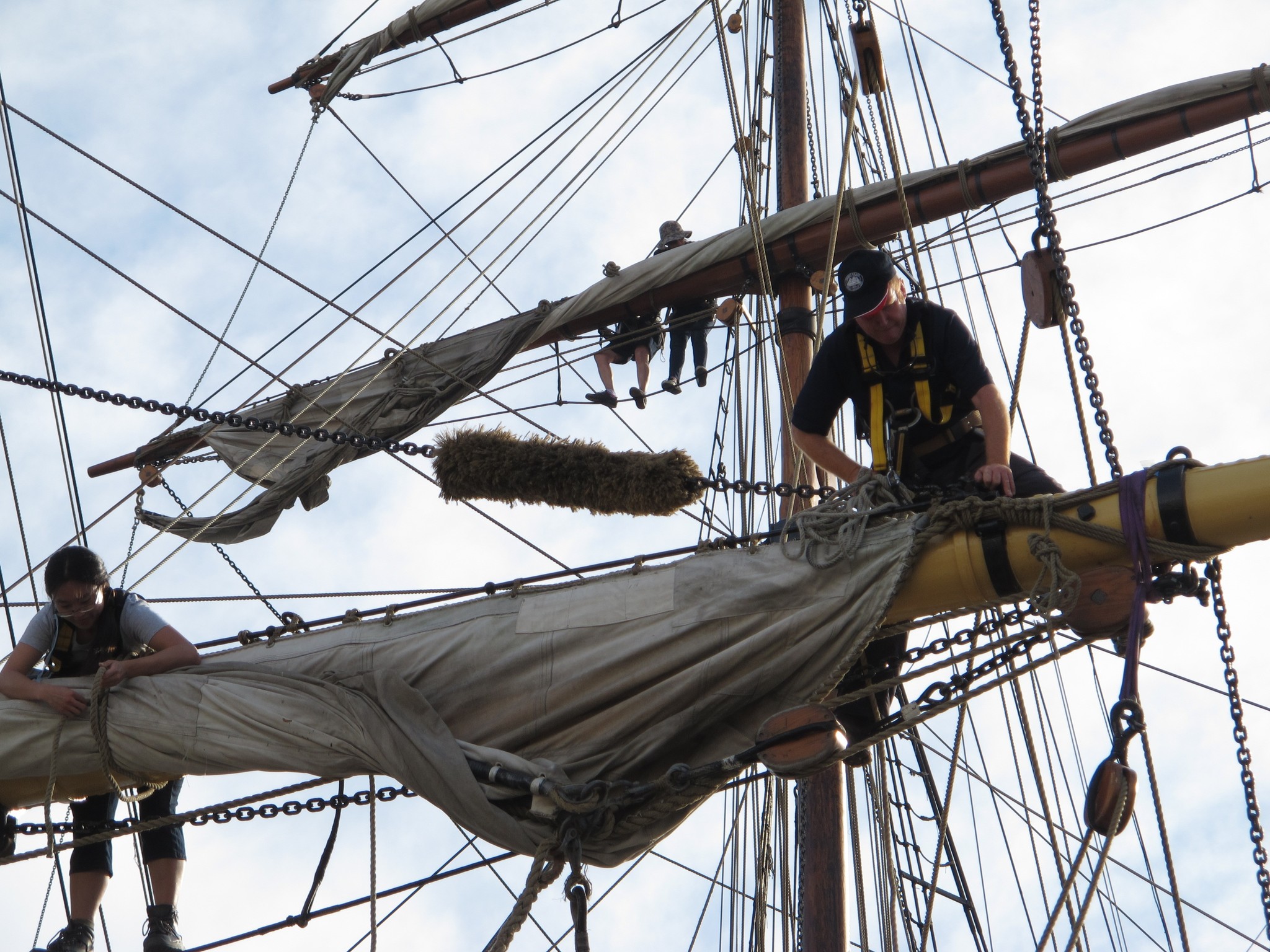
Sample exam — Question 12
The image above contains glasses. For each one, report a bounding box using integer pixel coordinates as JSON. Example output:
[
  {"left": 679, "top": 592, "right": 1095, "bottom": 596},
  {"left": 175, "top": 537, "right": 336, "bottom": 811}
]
[
  {"left": 50, "top": 584, "right": 99, "bottom": 618},
  {"left": 864, "top": 327, "right": 917, "bottom": 377}
]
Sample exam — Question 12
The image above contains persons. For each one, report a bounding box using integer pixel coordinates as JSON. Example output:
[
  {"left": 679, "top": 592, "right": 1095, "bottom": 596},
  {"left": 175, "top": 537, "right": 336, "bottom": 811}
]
[
  {"left": 1, "top": 544, "right": 202, "bottom": 952},
  {"left": 585, "top": 221, "right": 716, "bottom": 408},
  {"left": 791, "top": 246, "right": 1152, "bottom": 767}
]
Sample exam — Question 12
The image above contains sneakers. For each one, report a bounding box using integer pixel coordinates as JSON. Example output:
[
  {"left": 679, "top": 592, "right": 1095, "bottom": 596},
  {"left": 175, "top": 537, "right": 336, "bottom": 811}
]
[
  {"left": 47, "top": 922, "right": 95, "bottom": 952},
  {"left": 661, "top": 377, "right": 682, "bottom": 395},
  {"left": 585, "top": 390, "right": 618, "bottom": 408},
  {"left": 695, "top": 365, "right": 707, "bottom": 387},
  {"left": 629, "top": 387, "right": 647, "bottom": 409},
  {"left": 142, "top": 904, "right": 186, "bottom": 951}
]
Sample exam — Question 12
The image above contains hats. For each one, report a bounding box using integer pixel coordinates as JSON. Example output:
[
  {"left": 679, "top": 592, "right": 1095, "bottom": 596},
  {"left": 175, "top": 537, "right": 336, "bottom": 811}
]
[
  {"left": 838, "top": 249, "right": 896, "bottom": 323},
  {"left": 656, "top": 221, "right": 692, "bottom": 250}
]
